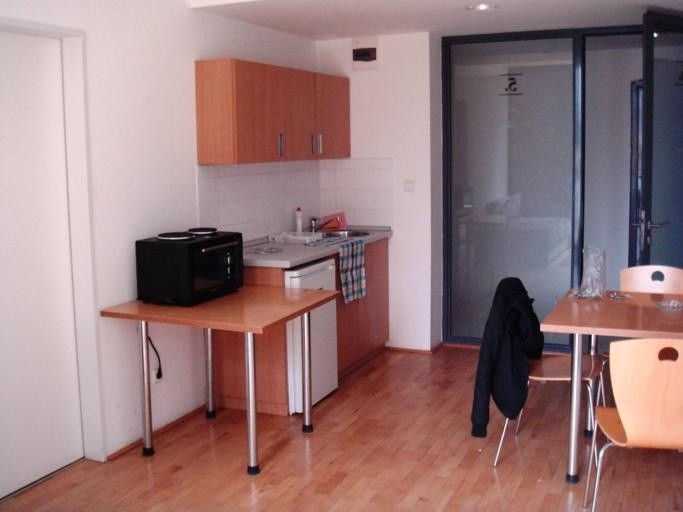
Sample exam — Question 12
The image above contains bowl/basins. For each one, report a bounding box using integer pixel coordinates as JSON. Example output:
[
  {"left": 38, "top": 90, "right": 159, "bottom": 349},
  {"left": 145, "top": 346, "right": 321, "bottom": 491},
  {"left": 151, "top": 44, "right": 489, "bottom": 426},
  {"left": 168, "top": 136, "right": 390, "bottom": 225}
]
[{"left": 656, "top": 299, "right": 683, "bottom": 314}]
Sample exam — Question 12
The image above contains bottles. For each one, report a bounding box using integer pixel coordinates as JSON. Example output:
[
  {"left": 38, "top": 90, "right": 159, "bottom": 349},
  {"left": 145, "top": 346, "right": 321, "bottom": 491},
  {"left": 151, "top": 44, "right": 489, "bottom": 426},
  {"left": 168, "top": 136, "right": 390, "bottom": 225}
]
[{"left": 294, "top": 207, "right": 304, "bottom": 233}]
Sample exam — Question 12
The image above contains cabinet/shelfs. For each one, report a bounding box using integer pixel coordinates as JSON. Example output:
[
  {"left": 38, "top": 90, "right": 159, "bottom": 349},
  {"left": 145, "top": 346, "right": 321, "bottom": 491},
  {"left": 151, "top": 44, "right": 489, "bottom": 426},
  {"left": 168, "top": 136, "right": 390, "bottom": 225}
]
[
  {"left": 197, "top": 58, "right": 285, "bottom": 166},
  {"left": 333, "top": 237, "right": 389, "bottom": 380},
  {"left": 284, "top": 67, "right": 350, "bottom": 162}
]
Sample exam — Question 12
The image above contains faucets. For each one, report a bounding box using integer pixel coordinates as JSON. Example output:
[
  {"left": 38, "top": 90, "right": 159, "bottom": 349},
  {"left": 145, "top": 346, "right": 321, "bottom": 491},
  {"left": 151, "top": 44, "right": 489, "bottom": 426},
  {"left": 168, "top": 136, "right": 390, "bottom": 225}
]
[{"left": 309, "top": 214, "right": 340, "bottom": 232}]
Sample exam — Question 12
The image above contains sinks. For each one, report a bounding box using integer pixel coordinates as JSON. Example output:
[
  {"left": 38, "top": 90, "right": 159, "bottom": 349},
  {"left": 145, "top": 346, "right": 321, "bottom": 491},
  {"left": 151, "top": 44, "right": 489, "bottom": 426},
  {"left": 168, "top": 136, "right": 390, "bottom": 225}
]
[{"left": 323, "top": 227, "right": 370, "bottom": 241}]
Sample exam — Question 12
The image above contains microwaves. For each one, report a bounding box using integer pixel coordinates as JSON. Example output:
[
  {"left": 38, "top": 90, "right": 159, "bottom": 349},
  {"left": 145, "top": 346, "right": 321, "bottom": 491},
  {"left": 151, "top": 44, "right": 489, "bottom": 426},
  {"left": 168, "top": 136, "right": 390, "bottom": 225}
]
[{"left": 136, "top": 231, "right": 246, "bottom": 306}]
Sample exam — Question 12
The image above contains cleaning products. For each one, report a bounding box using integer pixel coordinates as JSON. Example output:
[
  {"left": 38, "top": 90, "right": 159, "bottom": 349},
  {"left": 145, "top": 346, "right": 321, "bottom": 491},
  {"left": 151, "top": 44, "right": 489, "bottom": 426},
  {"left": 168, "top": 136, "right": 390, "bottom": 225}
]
[{"left": 295, "top": 204, "right": 303, "bottom": 233}]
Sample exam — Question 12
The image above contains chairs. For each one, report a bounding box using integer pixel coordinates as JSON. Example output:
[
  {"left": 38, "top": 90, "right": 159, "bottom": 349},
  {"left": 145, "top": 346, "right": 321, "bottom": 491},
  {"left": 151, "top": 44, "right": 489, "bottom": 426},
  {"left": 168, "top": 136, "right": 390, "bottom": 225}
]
[{"left": 491, "top": 264, "right": 683, "bottom": 511}]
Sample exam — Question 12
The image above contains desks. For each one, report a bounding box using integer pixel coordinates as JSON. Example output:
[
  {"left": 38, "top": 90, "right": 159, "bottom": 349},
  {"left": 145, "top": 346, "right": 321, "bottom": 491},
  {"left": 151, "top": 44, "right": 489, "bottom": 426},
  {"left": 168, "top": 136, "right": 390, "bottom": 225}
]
[{"left": 99, "top": 282, "right": 341, "bottom": 475}]
